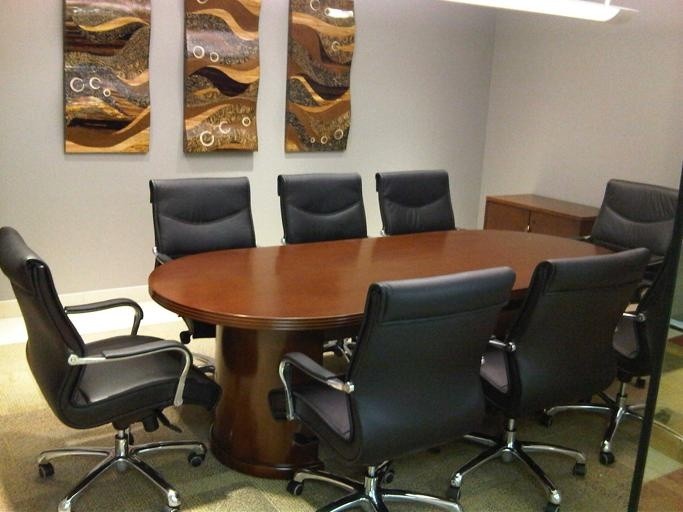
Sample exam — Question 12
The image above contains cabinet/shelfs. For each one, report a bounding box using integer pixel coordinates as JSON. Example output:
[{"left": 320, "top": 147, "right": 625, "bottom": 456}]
[{"left": 483, "top": 192, "right": 601, "bottom": 238}]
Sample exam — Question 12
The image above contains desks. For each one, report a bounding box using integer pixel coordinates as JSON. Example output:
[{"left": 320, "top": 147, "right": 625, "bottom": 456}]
[{"left": 149, "top": 229, "right": 620, "bottom": 481}]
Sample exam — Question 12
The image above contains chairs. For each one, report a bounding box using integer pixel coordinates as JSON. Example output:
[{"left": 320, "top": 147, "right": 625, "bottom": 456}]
[
  {"left": 588, "top": 179, "right": 679, "bottom": 314},
  {"left": 376, "top": 171, "right": 455, "bottom": 239},
  {"left": 265, "top": 264, "right": 518, "bottom": 512},
  {"left": 534, "top": 177, "right": 681, "bottom": 467},
  {"left": 431, "top": 247, "right": 655, "bottom": 512},
  {"left": 277, "top": 172, "right": 368, "bottom": 245},
  {"left": 148, "top": 175, "right": 257, "bottom": 343},
  {"left": 2, "top": 223, "right": 222, "bottom": 511}
]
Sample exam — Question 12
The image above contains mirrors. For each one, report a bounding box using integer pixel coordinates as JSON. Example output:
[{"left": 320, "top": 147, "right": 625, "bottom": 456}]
[{"left": 628, "top": 192, "right": 682, "bottom": 511}]
